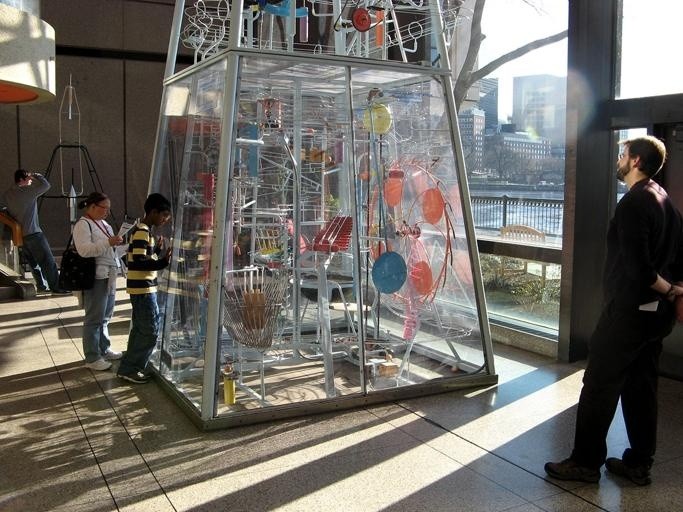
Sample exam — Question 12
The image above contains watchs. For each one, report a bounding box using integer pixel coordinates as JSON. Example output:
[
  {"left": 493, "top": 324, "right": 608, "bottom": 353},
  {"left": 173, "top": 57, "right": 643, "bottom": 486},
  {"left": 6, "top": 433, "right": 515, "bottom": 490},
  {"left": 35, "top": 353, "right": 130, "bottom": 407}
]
[{"left": 667, "top": 286, "right": 675, "bottom": 298}]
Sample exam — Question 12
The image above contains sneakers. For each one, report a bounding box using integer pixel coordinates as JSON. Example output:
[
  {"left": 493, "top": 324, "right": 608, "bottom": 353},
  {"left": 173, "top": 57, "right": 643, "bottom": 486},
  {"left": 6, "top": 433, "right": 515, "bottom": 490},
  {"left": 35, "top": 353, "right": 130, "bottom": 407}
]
[
  {"left": 86, "top": 359, "right": 113, "bottom": 370},
  {"left": 118, "top": 371, "right": 150, "bottom": 384},
  {"left": 606, "top": 457, "right": 652, "bottom": 486},
  {"left": 544, "top": 455, "right": 601, "bottom": 484},
  {"left": 103, "top": 350, "right": 122, "bottom": 361}
]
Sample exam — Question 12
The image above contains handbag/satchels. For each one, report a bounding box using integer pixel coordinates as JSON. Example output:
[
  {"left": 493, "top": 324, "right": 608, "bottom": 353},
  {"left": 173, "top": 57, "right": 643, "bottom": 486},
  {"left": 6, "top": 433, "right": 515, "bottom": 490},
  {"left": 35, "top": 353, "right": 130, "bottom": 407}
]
[{"left": 57, "top": 248, "right": 95, "bottom": 291}]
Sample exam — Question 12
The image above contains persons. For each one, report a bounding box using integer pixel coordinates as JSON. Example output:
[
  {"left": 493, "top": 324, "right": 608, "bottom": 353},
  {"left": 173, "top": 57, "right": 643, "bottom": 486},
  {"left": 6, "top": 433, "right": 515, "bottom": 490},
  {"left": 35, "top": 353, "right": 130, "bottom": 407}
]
[
  {"left": 72, "top": 193, "right": 123, "bottom": 371},
  {"left": 545, "top": 135, "right": 683, "bottom": 487},
  {"left": 116, "top": 193, "right": 173, "bottom": 384},
  {"left": 0, "top": 169, "right": 72, "bottom": 296}
]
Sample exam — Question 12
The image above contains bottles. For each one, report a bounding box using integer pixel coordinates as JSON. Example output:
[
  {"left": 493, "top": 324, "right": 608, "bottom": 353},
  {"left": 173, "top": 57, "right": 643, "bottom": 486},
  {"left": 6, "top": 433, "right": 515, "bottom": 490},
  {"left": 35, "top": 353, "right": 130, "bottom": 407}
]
[{"left": 223, "top": 365, "right": 235, "bottom": 406}]
